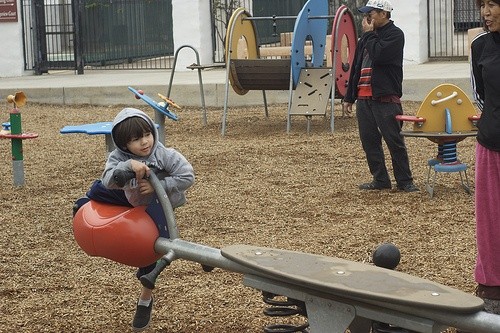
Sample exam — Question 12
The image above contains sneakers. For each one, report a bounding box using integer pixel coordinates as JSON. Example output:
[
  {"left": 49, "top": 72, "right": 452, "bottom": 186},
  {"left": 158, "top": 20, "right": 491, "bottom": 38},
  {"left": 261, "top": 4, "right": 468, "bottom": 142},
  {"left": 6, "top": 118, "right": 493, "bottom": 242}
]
[
  {"left": 397, "top": 184, "right": 419, "bottom": 192},
  {"left": 359, "top": 182, "right": 391, "bottom": 190}
]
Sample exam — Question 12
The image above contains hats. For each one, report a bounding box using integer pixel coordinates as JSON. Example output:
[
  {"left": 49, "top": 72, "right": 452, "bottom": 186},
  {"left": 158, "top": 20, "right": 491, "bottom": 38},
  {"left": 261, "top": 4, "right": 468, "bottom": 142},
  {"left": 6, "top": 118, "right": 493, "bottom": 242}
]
[{"left": 357, "top": 0, "right": 392, "bottom": 14}]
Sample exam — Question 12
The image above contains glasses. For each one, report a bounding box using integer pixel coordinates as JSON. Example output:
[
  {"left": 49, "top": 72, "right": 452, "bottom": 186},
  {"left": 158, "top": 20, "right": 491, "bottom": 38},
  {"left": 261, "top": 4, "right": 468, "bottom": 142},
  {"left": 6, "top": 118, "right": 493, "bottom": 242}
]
[{"left": 367, "top": 11, "right": 381, "bottom": 16}]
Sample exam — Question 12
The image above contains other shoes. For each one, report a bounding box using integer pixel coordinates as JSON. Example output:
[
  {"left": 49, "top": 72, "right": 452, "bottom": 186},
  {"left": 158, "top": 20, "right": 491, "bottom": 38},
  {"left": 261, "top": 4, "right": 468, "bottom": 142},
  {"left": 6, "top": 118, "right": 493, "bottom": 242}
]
[{"left": 133, "top": 295, "right": 153, "bottom": 328}]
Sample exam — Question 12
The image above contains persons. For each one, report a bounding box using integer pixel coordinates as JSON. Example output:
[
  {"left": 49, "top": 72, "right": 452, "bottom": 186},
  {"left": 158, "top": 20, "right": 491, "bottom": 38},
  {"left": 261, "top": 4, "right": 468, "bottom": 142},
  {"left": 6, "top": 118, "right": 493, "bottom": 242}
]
[
  {"left": 469, "top": 0, "right": 500, "bottom": 301},
  {"left": 344, "top": 0, "right": 416, "bottom": 193},
  {"left": 100, "top": 108, "right": 196, "bottom": 328}
]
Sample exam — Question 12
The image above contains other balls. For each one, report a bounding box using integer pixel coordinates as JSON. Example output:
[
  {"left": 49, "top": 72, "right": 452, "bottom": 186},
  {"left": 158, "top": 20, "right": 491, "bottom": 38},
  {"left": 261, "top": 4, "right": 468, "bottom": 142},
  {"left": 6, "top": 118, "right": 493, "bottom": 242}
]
[{"left": 373, "top": 242, "right": 400, "bottom": 270}]
